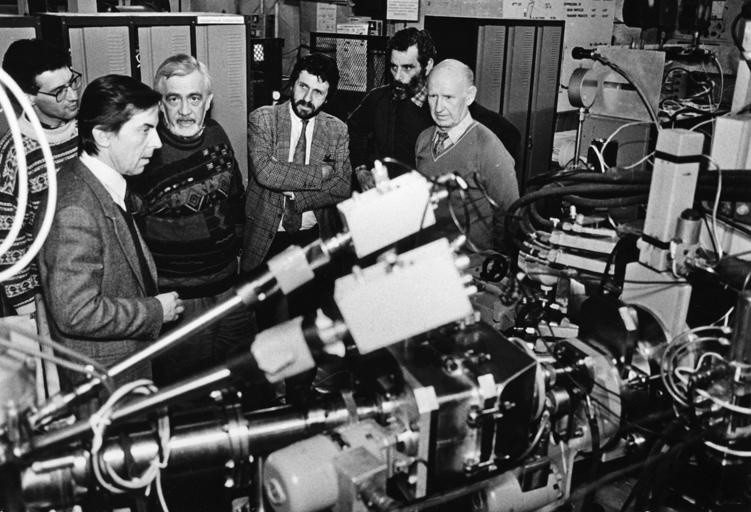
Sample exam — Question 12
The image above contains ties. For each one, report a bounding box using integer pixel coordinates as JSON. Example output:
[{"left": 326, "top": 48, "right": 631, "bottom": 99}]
[
  {"left": 434, "top": 131, "right": 450, "bottom": 159},
  {"left": 281, "top": 119, "right": 309, "bottom": 236}
]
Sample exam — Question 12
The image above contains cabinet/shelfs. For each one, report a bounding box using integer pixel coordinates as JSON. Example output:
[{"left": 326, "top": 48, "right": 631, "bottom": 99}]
[
  {"left": 0, "top": 9, "right": 252, "bottom": 190},
  {"left": 423, "top": 13, "right": 566, "bottom": 197}
]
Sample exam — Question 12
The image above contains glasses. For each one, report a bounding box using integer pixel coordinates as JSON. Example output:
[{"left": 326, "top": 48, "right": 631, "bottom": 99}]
[{"left": 37, "top": 69, "right": 85, "bottom": 103}]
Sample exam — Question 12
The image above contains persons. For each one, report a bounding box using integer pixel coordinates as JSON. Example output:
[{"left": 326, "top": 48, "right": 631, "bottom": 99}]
[
  {"left": 34, "top": 75, "right": 186, "bottom": 420},
  {"left": 415, "top": 59, "right": 521, "bottom": 250},
  {"left": 124, "top": 55, "right": 244, "bottom": 411},
  {"left": 345, "top": 27, "right": 521, "bottom": 197},
  {"left": 245, "top": 53, "right": 353, "bottom": 404},
  {"left": 2, "top": 38, "right": 84, "bottom": 314}
]
